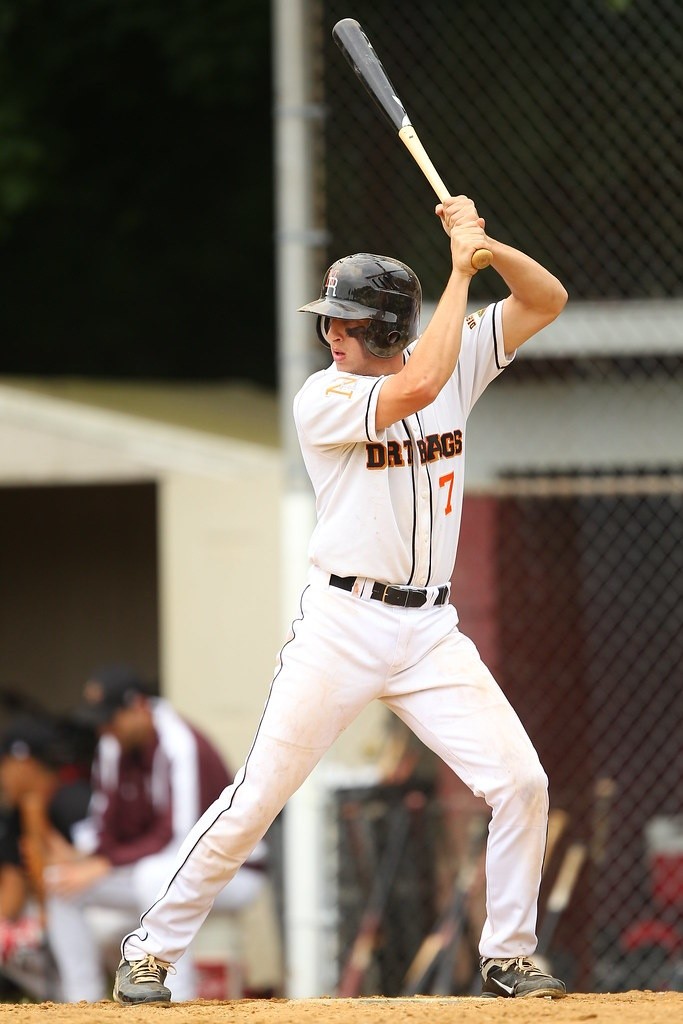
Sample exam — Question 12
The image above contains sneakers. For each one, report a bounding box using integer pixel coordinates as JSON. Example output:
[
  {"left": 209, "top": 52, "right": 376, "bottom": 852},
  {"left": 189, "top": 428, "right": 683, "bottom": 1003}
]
[
  {"left": 479, "top": 954, "right": 565, "bottom": 1001},
  {"left": 112, "top": 953, "right": 177, "bottom": 1007}
]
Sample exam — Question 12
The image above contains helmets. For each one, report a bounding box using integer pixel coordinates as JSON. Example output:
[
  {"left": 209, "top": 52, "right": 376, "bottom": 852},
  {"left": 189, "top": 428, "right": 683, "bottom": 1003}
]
[{"left": 295, "top": 254, "right": 422, "bottom": 360}]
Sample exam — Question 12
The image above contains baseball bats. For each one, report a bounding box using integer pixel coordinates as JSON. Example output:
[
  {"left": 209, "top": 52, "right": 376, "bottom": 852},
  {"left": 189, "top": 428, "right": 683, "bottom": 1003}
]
[{"left": 333, "top": 17, "right": 490, "bottom": 275}]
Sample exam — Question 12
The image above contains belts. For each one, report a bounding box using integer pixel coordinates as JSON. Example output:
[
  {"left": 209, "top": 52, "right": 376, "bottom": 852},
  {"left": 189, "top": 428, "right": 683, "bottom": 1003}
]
[{"left": 330, "top": 573, "right": 449, "bottom": 608}]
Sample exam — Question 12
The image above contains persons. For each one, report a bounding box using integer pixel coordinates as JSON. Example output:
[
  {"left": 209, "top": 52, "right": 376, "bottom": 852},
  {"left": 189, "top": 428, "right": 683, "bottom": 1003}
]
[
  {"left": 109, "top": 195, "right": 570, "bottom": 1005},
  {"left": 1, "top": 683, "right": 267, "bottom": 1007}
]
